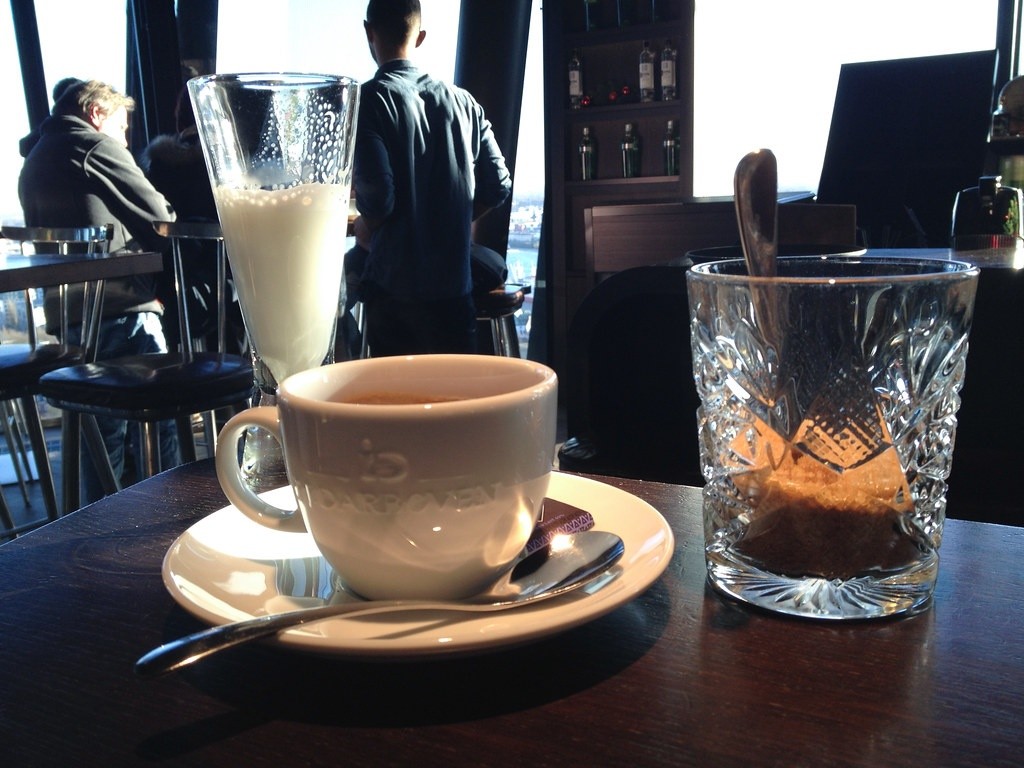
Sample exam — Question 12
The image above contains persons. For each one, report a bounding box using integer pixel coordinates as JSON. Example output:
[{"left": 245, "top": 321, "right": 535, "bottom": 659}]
[
  {"left": 53, "top": 66, "right": 248, "bottom": 356},
  {"left": 334, "top": 0, "right": 513, "bottom": 361},
  {"left": 18, "top": 82, "right": 181, "bottom": 486}
]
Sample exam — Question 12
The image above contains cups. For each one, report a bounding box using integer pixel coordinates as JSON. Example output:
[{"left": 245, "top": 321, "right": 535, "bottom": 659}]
[
  {"left": 215, "top": 355, "right": 558, "bottom": 602},
  {"left": 685, "top": 255, "right": 980, "bottom": 620},
  {"left": 186, "top": 73, "right": 362, "bottom": 495}
]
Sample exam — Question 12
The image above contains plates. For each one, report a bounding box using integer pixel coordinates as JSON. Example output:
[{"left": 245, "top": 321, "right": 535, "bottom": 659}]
[{"left": 162, "top": 471, "right": 675, "bottom": 657}]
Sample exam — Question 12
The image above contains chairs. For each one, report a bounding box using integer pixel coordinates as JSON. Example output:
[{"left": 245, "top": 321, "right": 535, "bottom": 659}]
[{"left": 558, "top": 204, "right": 858, "bottom": 470}]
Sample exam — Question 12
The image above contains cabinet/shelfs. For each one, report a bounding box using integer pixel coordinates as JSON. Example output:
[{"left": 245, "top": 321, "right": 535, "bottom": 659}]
[{"left": 544, "top": 0, "right": 695, "bottom": 400}]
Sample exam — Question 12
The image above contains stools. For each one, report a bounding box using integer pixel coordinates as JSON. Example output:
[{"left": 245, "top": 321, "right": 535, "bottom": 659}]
[{"left": 0, "top": 209, "right": 531, "bottom": 545}]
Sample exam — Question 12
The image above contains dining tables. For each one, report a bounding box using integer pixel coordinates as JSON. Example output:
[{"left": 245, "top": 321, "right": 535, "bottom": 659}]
[
  {"left": 0, "top": 252, "right": 164, "bottom": 293},
  {"left": 1, "top": 457, "right": 1024, "bottom": 768},
  {"left": 567, "top": 243, "right": 1024, "bottom": 527}
]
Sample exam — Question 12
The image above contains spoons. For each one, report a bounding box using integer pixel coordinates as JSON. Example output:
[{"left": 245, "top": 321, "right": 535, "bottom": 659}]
[{"left": 135, "top": 531, "right": 625, "bottom": 676}]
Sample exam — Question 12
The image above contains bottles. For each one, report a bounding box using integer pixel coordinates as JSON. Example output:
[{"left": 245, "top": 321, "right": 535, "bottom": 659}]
[
  {"left": 578, "top": 128, "right": 597, "bottom": 181},
  {"left": 992, "top": 96, "right": 1012, "bottom": 136},
  {"left": 661, "top": 120, "right": 680, "bottom": 176},
  {"left": 617, "top": 123, "right": 642, "bottom": 178},
  {"left": 568, "top": 39, "right": 677, "bottom": 105}
]
